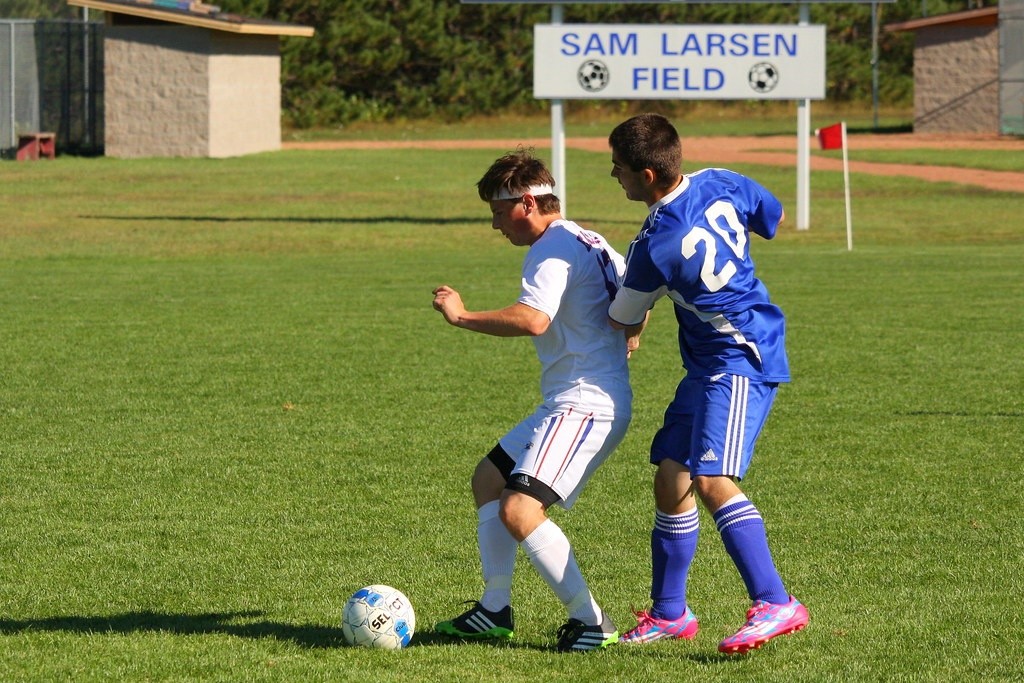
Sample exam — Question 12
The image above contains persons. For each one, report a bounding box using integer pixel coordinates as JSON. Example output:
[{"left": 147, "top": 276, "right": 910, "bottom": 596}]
[
  {"left": 607, "top": 116, "right": 810, "bottom": 655},
  {"left": 432, "top": 150, "right": 650, "bottom": 655}
]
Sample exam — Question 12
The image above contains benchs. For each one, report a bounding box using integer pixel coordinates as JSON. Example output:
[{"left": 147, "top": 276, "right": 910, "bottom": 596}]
[{"left": 15, "top": 131, "right": 57, "bottom": 160}]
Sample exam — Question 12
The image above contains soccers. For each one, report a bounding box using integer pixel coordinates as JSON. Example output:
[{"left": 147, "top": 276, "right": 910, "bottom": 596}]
[{"left": 343, "top": 584, "right": 418, "bottom": 648}]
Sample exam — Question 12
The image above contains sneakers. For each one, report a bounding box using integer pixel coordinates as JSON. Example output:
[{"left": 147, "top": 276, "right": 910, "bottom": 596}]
[
  {"left": 717, "top": 592, "right": 810, "bottom": 654},
  {"left": 434, "top": 600, "right": 516, "bottom": 642},
  {"left": 617, "top": 604, "right": 698, "bottom": 645},
  {"left": 552, "top": 609, "right": 619, "bottom": 653}
]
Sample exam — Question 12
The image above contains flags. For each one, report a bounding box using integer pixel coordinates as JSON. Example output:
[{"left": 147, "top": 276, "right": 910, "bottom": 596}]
[{"left": 815, "top": 122, "right": 845, "bottom": 152}]
